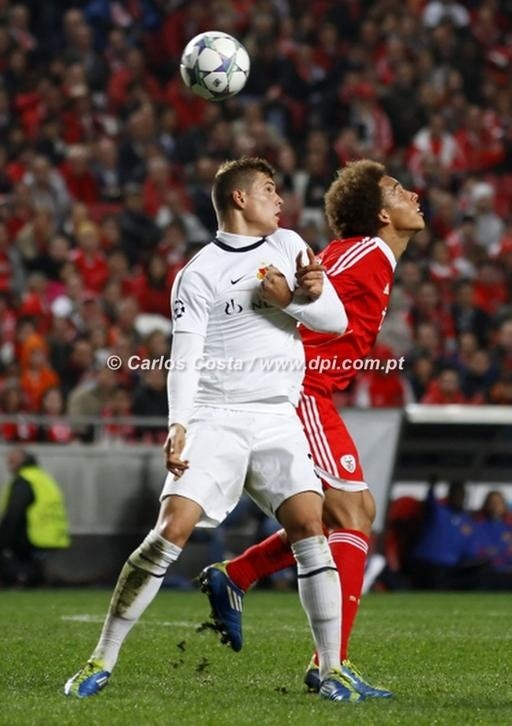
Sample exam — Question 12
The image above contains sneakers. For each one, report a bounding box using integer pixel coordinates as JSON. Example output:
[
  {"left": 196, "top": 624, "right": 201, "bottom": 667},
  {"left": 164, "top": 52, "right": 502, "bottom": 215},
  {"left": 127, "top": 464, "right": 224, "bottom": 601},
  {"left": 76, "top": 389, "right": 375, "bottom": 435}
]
[
  {"left": 194, "top": 560, "right": 246, "bottom": 652},
  {"left": 303, "top": 654, "right": 391, "bottom": 703},
  {"left": 64, "top": 657, "right": 111, "bottom": 699}
]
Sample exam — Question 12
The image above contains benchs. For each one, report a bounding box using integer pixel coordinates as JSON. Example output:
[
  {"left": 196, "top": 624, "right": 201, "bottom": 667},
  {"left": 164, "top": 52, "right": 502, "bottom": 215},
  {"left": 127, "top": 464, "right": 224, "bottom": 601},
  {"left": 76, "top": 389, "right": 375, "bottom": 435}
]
[{"left": 0, "top": 548, "right": 59, "bottom": 589}]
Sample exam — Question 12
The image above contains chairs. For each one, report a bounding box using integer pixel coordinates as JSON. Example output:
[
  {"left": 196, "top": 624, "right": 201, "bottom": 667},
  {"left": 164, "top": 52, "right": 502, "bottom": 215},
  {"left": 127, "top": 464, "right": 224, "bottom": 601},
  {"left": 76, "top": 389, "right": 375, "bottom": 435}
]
[{"left": 387, "top": 496, "right": 512, "bottom": 590}]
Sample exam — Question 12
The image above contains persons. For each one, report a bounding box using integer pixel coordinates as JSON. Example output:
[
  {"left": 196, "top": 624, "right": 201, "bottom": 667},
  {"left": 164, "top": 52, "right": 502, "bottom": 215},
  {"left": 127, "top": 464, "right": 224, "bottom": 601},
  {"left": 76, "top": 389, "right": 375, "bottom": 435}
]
[
  {"left": 191, "top": 157, "right": 427, "bottom": 698},
  {"left": 63, "top": 153, "right": 392, "bottom": 703},
  {"left": 0, "top": 0, "right": 511, "bottom": 590}
]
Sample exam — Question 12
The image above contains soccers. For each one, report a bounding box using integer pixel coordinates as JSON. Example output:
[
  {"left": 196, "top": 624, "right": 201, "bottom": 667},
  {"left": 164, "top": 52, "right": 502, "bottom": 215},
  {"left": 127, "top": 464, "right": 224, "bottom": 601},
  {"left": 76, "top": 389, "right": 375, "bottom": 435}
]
[{"left": 181, "top": 30, "right": 250, "bottom": 102}]
